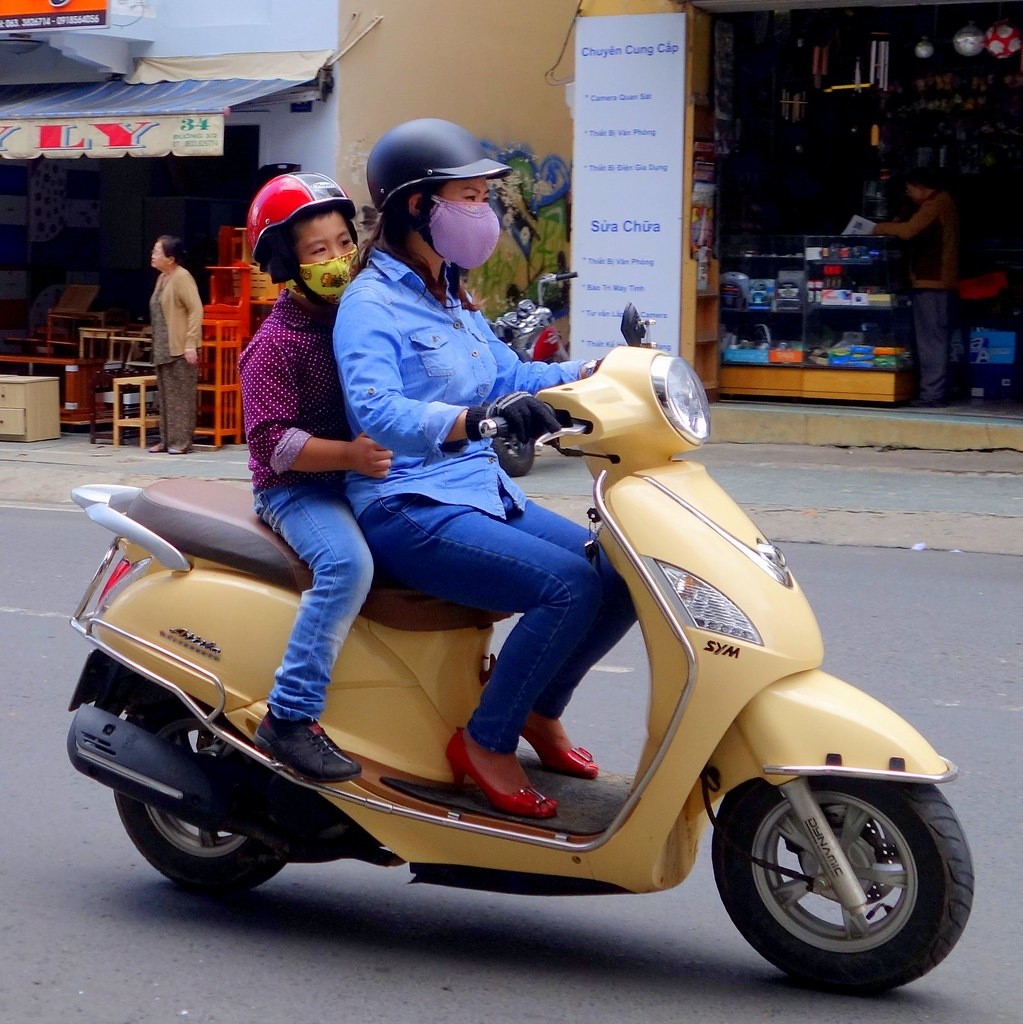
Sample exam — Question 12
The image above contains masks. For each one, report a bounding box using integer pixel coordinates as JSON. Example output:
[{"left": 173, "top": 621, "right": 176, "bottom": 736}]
[
  {"left": 429, "top": 195, "right": 500, "bottom": 269},
  {"left": 299, "top": 244, "right": 359, "bottom": 304}
]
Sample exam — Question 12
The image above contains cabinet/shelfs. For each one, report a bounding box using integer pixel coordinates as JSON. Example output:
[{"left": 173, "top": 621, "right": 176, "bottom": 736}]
[
  {"left": 192, "top": 320, "right": 242, "bottom": 448},
  {"left": 680, "top": 252, "right": 718, "bottom": 402},
  {"left": 0, "top": 309, "right": 160, "bottom": 448},
  {"left": 203, "top": 226, "right": 288, "bottom": 349},
  {"left": 717, "top": 234, "right": 920, "bottom": 403}
]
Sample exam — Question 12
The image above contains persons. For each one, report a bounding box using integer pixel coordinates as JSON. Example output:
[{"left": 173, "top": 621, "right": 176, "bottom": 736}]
[
  {"left": 334, "top": 118, "right": 638, "bottom": 818},
  {"left": 149, "top": 236, "right": 204, "bottom": 454},
  {"left": 238, "top": 172, "right": 393, "bottom": 783},
  {"left": 872, "top": 171, "right": 958, "bottom": 406}
]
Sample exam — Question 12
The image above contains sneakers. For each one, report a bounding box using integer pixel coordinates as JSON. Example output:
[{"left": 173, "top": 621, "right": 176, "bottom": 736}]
[{"left": 254, "top": 710, "right": 363, "bottom": 783}]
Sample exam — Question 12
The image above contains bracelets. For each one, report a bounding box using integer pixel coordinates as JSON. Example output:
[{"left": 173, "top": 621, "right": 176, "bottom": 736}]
[{"left": 586, "top": 359, "right": 599, "bottom": 377}]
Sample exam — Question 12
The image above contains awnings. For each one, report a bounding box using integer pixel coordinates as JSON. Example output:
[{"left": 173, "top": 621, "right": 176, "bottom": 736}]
[{"left": 0, "top": 58, "right": 318, "bottom": 157}]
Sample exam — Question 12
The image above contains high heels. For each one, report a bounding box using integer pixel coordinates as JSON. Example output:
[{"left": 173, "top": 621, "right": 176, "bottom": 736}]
[
  {"left": 445, "top": 727, "right": 558, "bottom": 818},
  {"left": 520, "top": 713, "right": 599, "bottom": 779}
]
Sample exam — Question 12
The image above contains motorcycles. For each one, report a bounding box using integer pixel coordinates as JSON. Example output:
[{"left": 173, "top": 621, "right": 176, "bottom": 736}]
[{"left": 478, "top": 269, "right": 578, "bottom": 479}]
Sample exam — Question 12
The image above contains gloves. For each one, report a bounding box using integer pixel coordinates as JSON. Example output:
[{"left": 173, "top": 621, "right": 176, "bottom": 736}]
[{"left": 465, "top": 391, "right": 561, "bottom": 444}]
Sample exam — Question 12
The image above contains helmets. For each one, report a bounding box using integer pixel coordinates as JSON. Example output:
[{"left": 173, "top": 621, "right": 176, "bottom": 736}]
[
  {"left": 247, "top": 171, "right": 357, "bottom": 265},
  {"left": 366, "top": 117, "right": 513, "bottom": 212}
]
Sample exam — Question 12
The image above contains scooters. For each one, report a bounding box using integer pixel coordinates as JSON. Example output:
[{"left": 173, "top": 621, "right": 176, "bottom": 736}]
[{"left": 63, "top": 296, "right": 976, "bottom": 1003}]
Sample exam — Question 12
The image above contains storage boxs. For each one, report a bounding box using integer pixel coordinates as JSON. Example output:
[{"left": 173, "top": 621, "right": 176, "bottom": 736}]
[
  {"left": 969, "top": 364, "right": 1015, "bottom": 400},
  {"left": 724, "top": 348, "right": 768, "bottom": 362},
  {"left": 767, "top": 348, "right": 802, "bottom": 362},
  {"left": 968, "top": 329, "right": 1015, "bottom": 364},
  {"left": 821, "top": 290, "right": 894, "bottom": 306}
]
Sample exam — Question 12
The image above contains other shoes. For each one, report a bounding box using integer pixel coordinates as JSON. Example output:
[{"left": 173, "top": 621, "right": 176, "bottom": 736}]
[
  {"left": 147, "top": 442, "right": 167, "bottom": 453},
  {"left": 168, "top": 448, "right": 185, "bottom": 454},
  {"left": 909, "top": 400, "right": 952, "bottom": 408}
]
